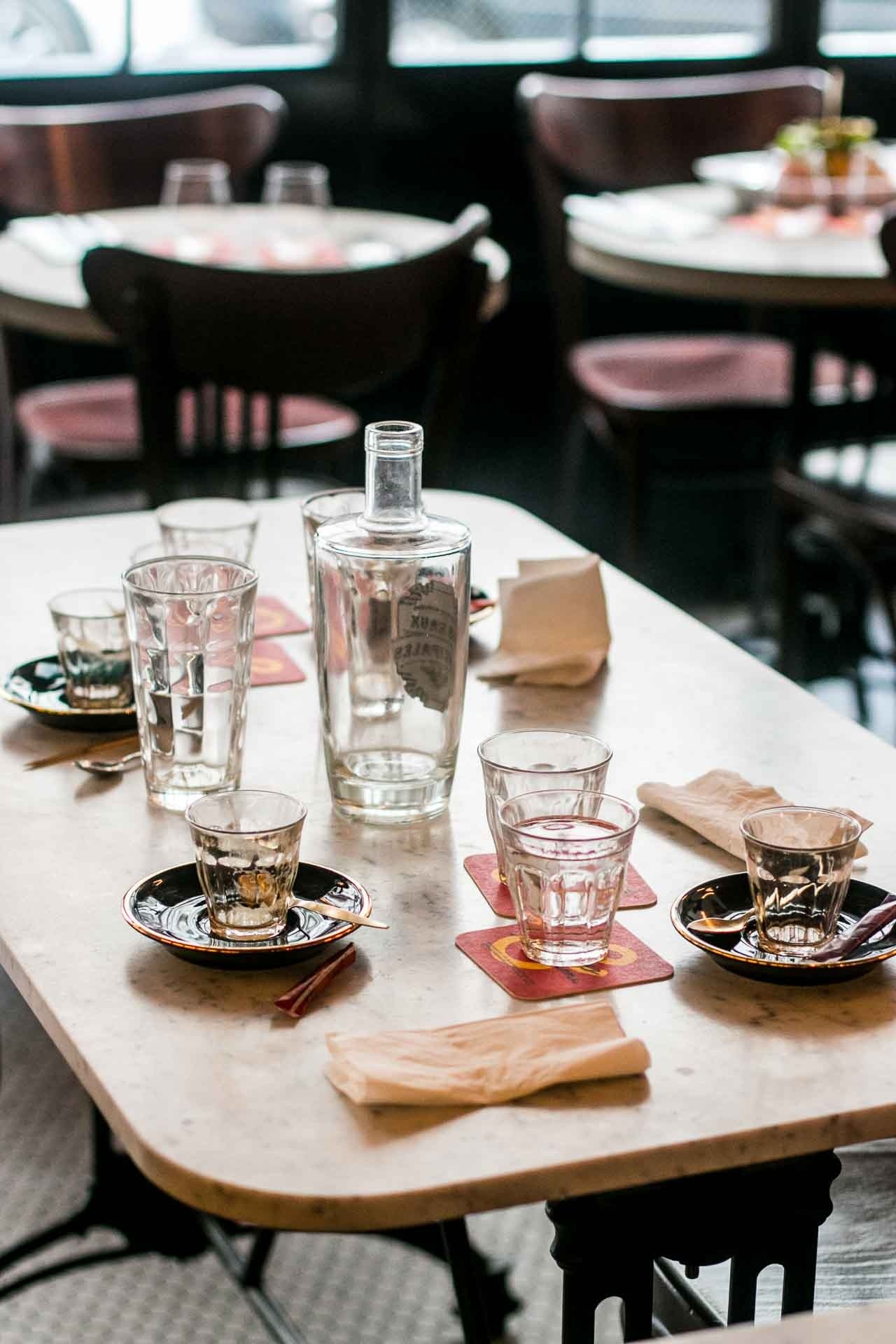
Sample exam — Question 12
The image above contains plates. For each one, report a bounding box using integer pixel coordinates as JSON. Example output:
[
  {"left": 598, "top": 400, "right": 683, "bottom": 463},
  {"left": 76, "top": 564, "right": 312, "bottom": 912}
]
[
  {"left": 0, "top": 652, "right": 140, "bottom": 734},
  {"left": 469, "top": 582, "right": 493, "bottom": 626},
  {"left": 694, "top": 148, "right": 894, "bottom": 208},
  {"left": 670, "top": 872, "right": 896, "bottom": 986},
  {"left": 121, "top": 861, "right": 372, "bottom": 970}
]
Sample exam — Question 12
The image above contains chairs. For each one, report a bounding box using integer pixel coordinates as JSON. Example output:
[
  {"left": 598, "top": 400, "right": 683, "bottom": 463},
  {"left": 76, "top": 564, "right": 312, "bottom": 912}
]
[
  {"left": 0, "top": 87, "right": 359, "bottom": 498},
  {"left": 514, "top": 64, "right": 877, "bottom": 630},
  {"left": 774, "top": 215, "right": 895, "bottom": 733},
  {"left": 79, "top": 204, "right": 496, "bottom": 514}
]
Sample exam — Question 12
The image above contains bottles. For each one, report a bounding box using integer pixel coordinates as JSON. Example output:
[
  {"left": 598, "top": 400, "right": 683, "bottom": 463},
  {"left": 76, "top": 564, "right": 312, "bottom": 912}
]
[{"left": 312, "top": 421, "right": 471, "bottom": 825}]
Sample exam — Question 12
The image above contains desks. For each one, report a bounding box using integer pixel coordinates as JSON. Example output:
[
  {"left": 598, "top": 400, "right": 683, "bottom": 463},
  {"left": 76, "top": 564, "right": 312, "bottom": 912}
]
[
  {"left": 568, "top": 181, "right": 895, "bottom": 676},
  {"left": 1, "top": 204, "right": 517, "bottom": 454},
  {"left": 2, "top": 486, "right": 895, "bottom": 1344}
]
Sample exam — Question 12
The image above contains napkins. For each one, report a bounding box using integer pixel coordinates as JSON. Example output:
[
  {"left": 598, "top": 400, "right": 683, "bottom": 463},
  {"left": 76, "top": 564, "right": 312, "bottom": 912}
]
[
  {"left": 476, "top": 551, "right": 613, "bottom": 685},
  {"left": 324, "top": 1004, "right": 652, "bottom": 1107},
  {"left": 634, "top": 769, "right": 871, "bottom": 864}
]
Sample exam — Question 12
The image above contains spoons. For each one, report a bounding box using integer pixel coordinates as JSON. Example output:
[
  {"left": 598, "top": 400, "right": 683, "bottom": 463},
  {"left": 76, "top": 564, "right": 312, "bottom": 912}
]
[
  {"left": 73, "top": 750, "right": 142, "bottom": 777},
  {"left": 239, "top": 875, "right": 390, "bottom": 929},
  {"left": 687, "top": 907, "right": 756, "bottom": 935}
]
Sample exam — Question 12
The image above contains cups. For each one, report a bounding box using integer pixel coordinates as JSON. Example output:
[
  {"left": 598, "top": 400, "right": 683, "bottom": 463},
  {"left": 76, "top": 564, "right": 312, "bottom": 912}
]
[
  {"left": 302, "top": 488, "right": 367, "bottom": 606},
  {"left": 157, "top": 158, "right": 233, "bottom": 265},
  {"left": 46, "top": 587, "right": 133, "bottom": 710},
  {"left": 154, "top": 497, "right": 260, "bottom": 567},
  {"left": 740, "top": 806, "right": 862, "bottom": 957},
  {"left": 258, "top": 161, "right": 341, "bottom": 273},
  {"left": 498, "top": 789, "right": 640, "bottom": 967},
  {"left": 121, "top": 555, "right": 259, "bottom": 813},
  {"left": 185, "top": 789, "right": 308, "bottom": 942},
  {"left": 477, "top": 728, "right": 613, "bottom": 886}
]
